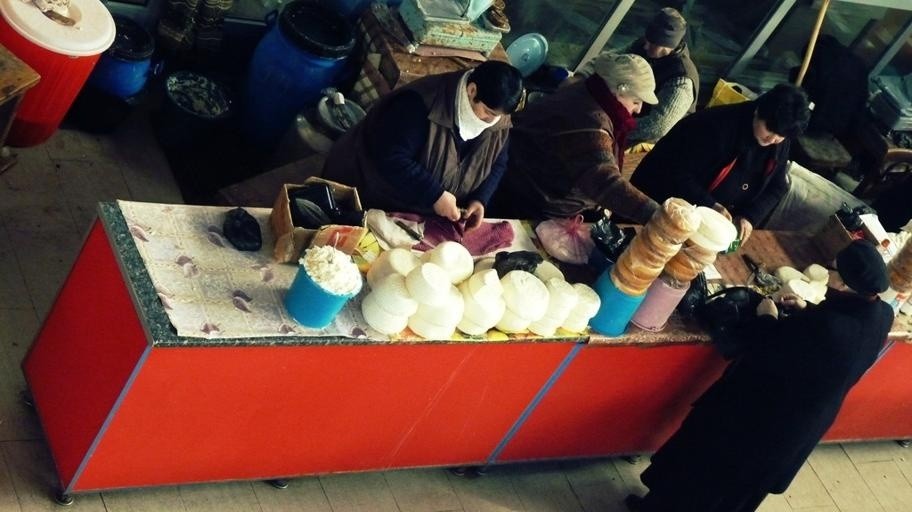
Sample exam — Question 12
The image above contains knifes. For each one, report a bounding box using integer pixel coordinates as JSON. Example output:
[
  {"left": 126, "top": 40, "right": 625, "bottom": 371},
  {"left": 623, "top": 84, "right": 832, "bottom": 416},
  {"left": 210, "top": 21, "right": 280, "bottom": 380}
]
[{"left": 395, "top": 219, "right": 436, "bottom": 250}]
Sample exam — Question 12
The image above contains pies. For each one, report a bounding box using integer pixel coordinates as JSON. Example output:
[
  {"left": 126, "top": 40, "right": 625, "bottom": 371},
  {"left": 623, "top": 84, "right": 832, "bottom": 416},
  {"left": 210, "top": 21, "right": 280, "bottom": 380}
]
[{"left": 609, "top": 196, "right": 716, "bottom": 298}]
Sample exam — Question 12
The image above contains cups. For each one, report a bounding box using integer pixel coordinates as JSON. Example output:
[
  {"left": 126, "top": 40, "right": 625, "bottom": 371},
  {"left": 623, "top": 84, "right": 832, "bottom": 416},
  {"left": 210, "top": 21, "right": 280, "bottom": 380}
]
[{"left": 491, "top": 1, "right": 506, "bottom": 29}]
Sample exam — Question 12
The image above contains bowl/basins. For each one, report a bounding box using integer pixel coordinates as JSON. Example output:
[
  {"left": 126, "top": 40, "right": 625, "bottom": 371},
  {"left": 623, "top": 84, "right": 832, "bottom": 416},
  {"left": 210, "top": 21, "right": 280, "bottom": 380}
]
[
  {"left": 166, "top": 72, "right": 230, "bottom": 119},
  {"left": 361, "top": 241, "right": 602, "bottom": 337}
]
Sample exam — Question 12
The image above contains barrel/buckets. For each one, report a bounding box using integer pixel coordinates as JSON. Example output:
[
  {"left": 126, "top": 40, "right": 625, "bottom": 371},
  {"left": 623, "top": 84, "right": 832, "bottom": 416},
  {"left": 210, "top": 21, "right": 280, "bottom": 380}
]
[
  {"left": 2, "top": 0, "right": 117, "bottom": 150},
  {"left": 285, "top": 246, "right": 363, "bottom": 331},
  {"left": 73, "top": 10, "right": 154, "bottom": 134},
  {"left": 239, "top": 3, "right": 364, "bottom": 138},
  {"left": 159, "top": 69, "right": 238, "bottom": 144}
]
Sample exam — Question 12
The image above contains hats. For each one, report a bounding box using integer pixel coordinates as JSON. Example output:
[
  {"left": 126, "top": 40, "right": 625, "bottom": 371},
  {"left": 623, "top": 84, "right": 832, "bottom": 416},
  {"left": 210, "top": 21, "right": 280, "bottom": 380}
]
[
  {"left": 646, "top": 8, "right": 687, "bottom": 48},
  {"left": 837, "top": 239, "right": 889, "bottom": 294},
  {"left": 608, "top": 54, "right": 659, "bottom": 105}
]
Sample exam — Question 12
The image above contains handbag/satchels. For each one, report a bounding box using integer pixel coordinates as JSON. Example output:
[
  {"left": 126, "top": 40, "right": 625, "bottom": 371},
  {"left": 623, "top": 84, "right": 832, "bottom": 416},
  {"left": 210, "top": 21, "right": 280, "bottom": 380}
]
[{"left": 679, "top": 272, "right": 783, "bottom": 338}]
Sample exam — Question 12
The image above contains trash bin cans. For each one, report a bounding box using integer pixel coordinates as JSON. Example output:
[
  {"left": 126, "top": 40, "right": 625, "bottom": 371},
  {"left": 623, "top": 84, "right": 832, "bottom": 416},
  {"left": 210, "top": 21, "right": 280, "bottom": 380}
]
[{"left": 705, "top": 78, "right": 759, "bottom": 110}]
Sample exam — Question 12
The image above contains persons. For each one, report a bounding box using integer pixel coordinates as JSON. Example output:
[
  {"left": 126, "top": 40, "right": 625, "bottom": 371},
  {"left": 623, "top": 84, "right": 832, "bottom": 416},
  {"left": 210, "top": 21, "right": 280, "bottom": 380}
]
[
  {"left": 321, "top": 58, "right": 527, "bottom": 235},
  {"left": 484, "top": 48, "right": 664, "bottom": 228},
  {"left": 608, "top": 80, "right": 814, "bottom": 248},
  {"left": 556, "top": 5, "right": 703, "bottom": 156},
  {"left": 623, "top": 239, "right": 894, "bottom": 512}
]
